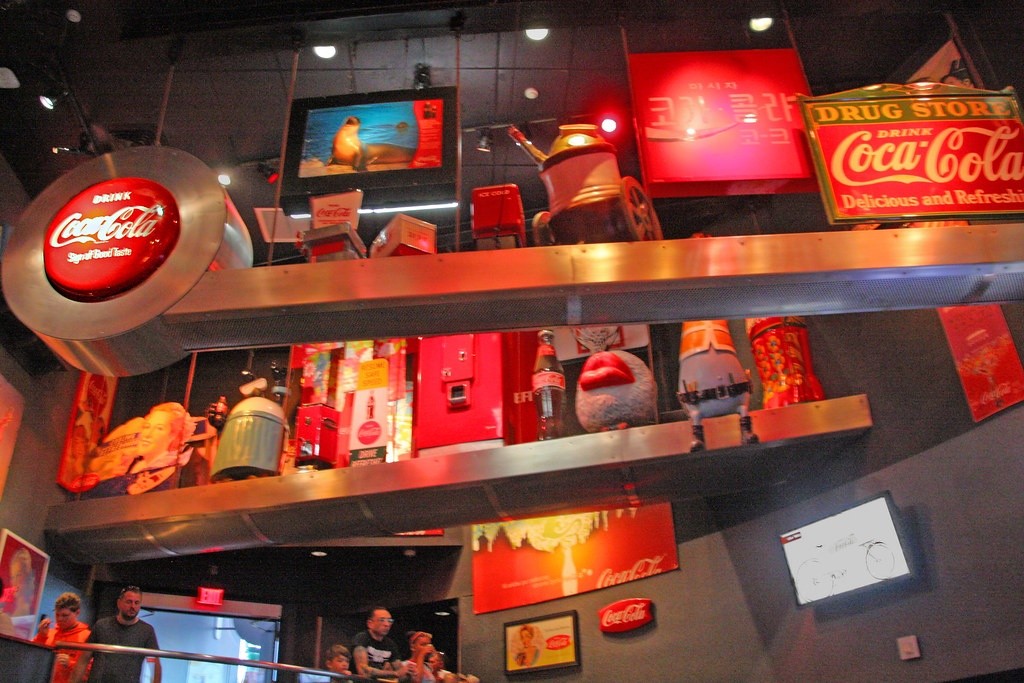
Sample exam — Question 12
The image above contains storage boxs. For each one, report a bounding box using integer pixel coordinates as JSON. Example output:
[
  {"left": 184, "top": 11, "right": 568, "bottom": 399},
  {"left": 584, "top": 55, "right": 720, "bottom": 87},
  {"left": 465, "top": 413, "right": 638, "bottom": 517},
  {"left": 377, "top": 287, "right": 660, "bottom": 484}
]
[
  {"left": 471, "top": 184, "right": 526, "bottom": 248},
  {"left": 303, "top": 220, "right": 366, "bottom": 264},
  {"left": 371, "top": 214, "right": 437, "bottom": 259},
  {"left": 278, "top": 336, "right": 417, "bottom": 475}
]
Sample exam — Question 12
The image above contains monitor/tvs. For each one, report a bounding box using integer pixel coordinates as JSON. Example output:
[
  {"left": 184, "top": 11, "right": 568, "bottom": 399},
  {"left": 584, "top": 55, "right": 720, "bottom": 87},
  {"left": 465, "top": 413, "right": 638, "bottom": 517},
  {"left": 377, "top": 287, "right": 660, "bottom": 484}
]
[
  {"left": 777, "top": 488, "right": 920, "bottom": 609},
  {"left": 281, "top": 84, "right": 459, "bottom": 197}
]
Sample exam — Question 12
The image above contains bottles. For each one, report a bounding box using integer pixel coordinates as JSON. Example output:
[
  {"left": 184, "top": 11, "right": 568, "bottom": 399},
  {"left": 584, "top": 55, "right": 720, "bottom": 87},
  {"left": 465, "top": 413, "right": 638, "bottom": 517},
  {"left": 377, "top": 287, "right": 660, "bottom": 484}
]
[{"left": 531, "top": 330, "right": 564, "bottom": 441}]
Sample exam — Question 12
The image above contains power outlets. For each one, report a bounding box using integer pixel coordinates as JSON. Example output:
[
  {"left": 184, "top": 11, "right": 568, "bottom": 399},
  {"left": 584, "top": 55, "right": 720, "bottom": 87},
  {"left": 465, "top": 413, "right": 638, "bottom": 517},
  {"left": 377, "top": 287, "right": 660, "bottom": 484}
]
[{"left": 897, "top": 636, "right": 921, "bottom": 660}]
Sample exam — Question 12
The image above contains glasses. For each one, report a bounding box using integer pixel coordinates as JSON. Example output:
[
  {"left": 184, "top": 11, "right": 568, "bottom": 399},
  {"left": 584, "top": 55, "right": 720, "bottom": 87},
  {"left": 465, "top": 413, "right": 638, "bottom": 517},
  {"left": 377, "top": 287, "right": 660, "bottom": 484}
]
[
  {"left": 370, "top": 619, "right": 394, "bottom": 625},
  {"left": 44, "top": 608, "right": 72, "bottom": 628},
  {"left": 120, "top": 586, "right": 140, "bottom": 595}
]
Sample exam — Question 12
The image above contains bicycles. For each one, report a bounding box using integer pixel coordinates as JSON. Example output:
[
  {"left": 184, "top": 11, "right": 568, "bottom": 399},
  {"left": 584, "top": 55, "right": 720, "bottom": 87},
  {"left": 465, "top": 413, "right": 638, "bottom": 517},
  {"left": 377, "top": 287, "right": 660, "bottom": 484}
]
[{"left": 794, "top": 532, "right": 895, "bottom": 602}]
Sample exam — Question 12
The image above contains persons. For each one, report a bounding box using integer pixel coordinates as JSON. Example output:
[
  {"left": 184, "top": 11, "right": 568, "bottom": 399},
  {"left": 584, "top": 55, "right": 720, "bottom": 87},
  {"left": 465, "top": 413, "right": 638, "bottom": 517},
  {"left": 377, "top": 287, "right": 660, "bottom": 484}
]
[
  {"left": 137, "top": 402, "right": 194, "bottom": 467},
  {"left": 352, "top": 606, "right": 419, "bottom": 683},
  {"left": 69, "top": 586, "right": 163, "bottom": 683},
  {"left": 324, "top": 645, "right": 354, "bottom": 683},
  {"left": 0, "top": 578, "right": 13, "bottom": 635},
  {"left": 28, "top": 592, "right": 94, "bottom": 683},
  {"left": 401, "top": 632, "right": 452, "bottom": 683}
]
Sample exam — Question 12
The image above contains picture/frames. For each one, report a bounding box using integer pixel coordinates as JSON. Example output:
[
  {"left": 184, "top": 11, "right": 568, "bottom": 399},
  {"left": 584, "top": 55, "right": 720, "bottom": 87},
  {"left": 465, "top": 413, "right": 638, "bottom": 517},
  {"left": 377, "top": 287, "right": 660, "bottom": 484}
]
[
  {"left": 503, "top": 610, "right": 581, "bottom": 675},
  {"left": 0, "top": 527, "right": 50, "bottom": 640}
]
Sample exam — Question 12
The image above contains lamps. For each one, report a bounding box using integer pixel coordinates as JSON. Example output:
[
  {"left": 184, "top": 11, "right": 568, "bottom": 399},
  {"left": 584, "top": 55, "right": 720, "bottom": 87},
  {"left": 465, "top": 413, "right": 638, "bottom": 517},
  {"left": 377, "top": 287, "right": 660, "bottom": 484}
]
[
  {"left": 40, "top": 82, "right": 68, "bottom": 109},
  {"left": 242, "top": 352, "right": 254, "bottom": 375},
  {"left": 52, "top": 134, "right": 93, "bottom": 157}
]
